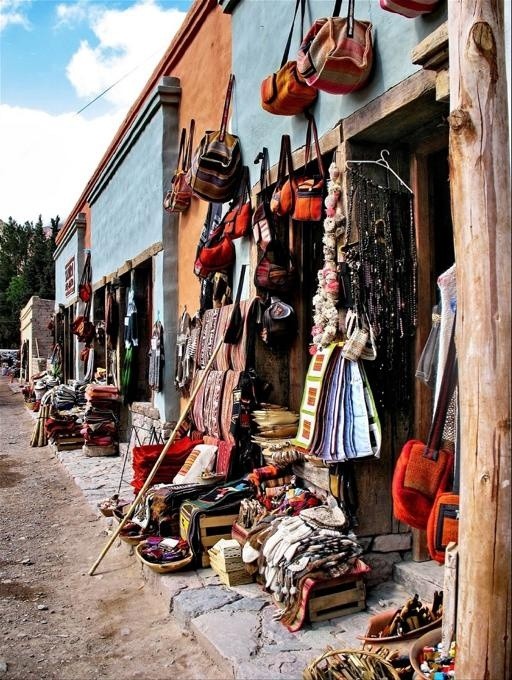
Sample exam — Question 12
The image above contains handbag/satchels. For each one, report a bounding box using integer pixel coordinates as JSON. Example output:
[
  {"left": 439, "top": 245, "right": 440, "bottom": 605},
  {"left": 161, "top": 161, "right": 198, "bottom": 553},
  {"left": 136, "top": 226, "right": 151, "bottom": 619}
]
[
  {"left": 184, "top": 128, "right": 244, "bottom": 204},
  {"left": 163, "top": 165, "right": 192, "bottom": 213},
  {"left": 298, "top": 15, "right": 376, "bottom": 96},
  {"left": 424, "top": 490, "right": 458, "bottom": 565},
  {"left": 195, "top": 178, "right": 325, "bottom": 293},
  {"left": 392, "top": 440, "right": 453, "bottom": 532},
  {"left": 70, "top": 281, "right": 94, "bottom": 363},
  {"left": 221, "top": 306, "right": 243, "bottom": 345},
  {"left": 260, "top": 61, "right": 317, "bottom": 117},
  {"left": 413, "top": 300, "right": 442, "bottom": 391},
  {"left": 378, "top": 0, "right": 442, "bottom": 18}
]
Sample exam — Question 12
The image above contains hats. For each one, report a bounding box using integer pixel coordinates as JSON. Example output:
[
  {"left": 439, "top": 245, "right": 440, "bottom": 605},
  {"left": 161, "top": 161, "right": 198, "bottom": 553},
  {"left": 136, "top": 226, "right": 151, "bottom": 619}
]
[{"left": 34, "top": 380, "right": 47, "bottom": 390}]
